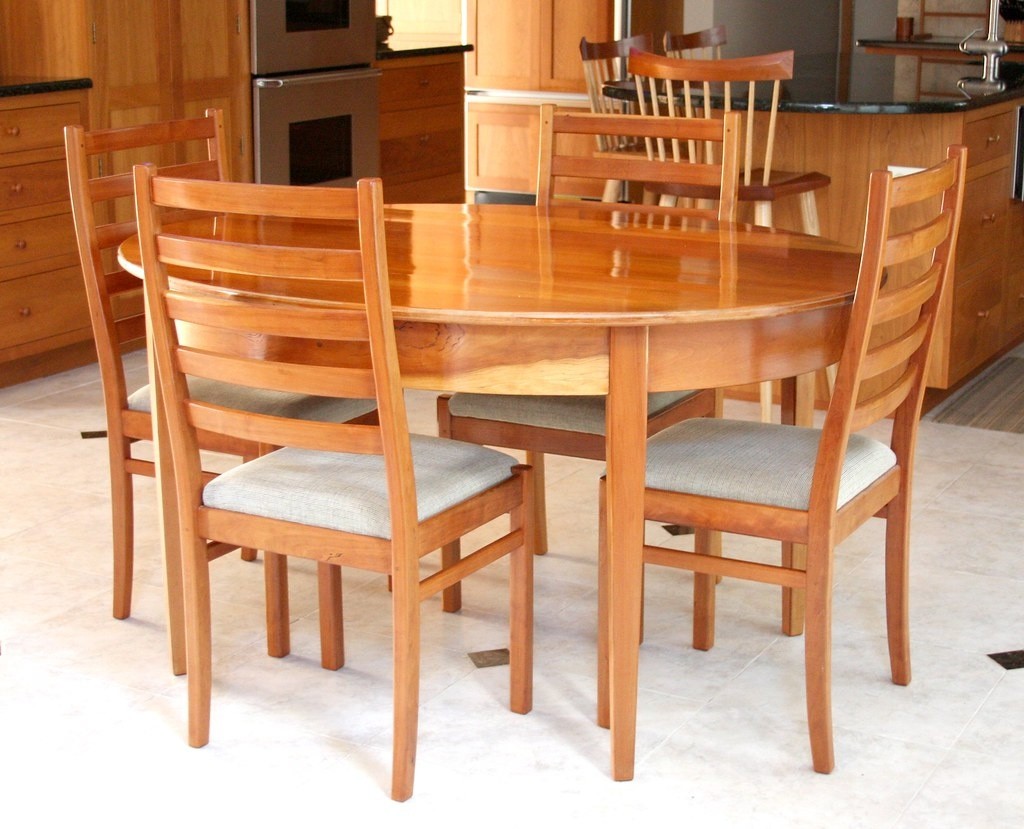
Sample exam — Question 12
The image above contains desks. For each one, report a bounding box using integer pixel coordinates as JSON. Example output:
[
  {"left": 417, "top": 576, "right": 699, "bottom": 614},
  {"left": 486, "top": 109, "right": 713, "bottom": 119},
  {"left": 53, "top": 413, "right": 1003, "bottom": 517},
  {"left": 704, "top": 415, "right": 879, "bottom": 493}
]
[{"left": 116, "top": 205, "right": 890, "bottom": 781}]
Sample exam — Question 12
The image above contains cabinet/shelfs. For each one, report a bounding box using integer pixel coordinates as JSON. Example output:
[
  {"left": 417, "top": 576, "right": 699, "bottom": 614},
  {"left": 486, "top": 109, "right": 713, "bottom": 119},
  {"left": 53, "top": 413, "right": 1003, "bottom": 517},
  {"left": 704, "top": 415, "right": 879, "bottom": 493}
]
[
  {"left": 690, "top": 98, "right": 1023, "bottom": 389},
  {"left": 0, "top": 80, "right": 96, "bottom": 371},
  {"left": 376, "top": 45, "right": 468, "bottom": 204}
]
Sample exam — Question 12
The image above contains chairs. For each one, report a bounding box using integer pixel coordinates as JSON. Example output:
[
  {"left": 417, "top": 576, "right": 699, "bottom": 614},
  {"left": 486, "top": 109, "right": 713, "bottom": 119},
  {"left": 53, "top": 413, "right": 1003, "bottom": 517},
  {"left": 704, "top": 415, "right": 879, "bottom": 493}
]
[{"left": 63, "top": 37, "right": 970, "bottom": 802}]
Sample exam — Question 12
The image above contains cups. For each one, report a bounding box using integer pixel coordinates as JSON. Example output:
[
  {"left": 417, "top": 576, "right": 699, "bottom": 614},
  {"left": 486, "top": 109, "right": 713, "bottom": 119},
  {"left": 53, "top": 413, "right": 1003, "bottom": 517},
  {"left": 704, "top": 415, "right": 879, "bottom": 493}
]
[{"left": 896, "top": 16, "right": 913, "bottom": 41}]
